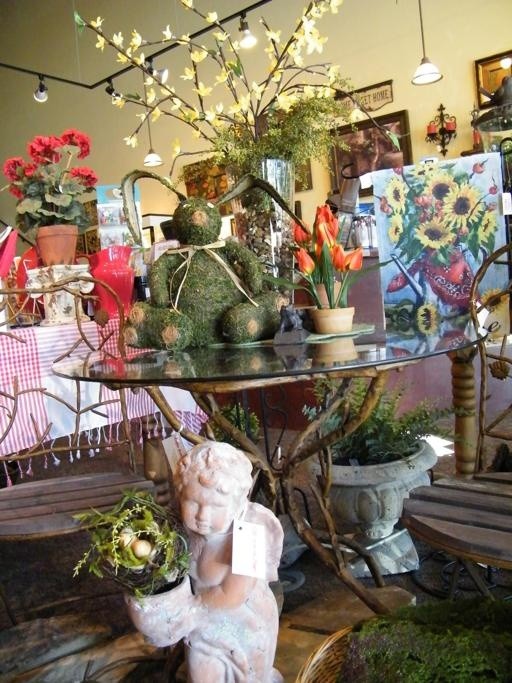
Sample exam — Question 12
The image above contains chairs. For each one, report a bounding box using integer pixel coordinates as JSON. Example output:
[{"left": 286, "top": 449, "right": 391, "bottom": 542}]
[
  {"left": 2, "top": 269, "right": 163, "bottom": 624},
  {"left": 401, "top": 237, "right": 512, "bottom": 578}
]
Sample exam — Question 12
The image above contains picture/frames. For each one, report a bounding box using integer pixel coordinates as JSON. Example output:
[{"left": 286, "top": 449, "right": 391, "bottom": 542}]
[
  {"left": 321, "top": 111, "right": 413, "bottom": 198},
  {"left": 470, "top": 47, "right": 512, "bottom": 108}
]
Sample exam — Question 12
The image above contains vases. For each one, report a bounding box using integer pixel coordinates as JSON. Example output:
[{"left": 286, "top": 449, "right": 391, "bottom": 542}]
[
  {"left": 311, "top": 304, "right": 356, "bottom": 334},
  {"left": 238, "top": 153, "right": 298, "bottom": 232},
  {"left": 35, "top": 221, "right": 81, "bottom": 267}
]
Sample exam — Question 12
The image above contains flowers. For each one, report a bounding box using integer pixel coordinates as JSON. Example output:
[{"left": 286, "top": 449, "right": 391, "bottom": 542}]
[
  {"left": 69, "top": 0, "right": 405, "bottom": 177},
  {"left": 268, "top": 204, "right": 396, "bottom": 310},
  {"left": 0, "top": 124, "right": 101, "bottom": 232}
]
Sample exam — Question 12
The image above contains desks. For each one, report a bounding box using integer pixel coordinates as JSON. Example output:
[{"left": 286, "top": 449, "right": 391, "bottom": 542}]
[
  {"left": 1, "top": 306, "right": 222, "bottom": 498},
  {"left": 60, "top": 320, "right": 483, "bottom": 619}
]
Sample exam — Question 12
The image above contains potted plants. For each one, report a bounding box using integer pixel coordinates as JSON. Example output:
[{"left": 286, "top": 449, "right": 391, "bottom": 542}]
[
  {"left": 76, "top": 486, "right": 197, "bottom": 654},
  {"left": 298, "top": 375, "right": 482, "bottom": 547}
]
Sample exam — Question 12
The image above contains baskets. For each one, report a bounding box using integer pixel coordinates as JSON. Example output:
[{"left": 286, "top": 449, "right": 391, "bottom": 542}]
[{"left": 294, "top": 623, "right": 354, "bottom": 682}]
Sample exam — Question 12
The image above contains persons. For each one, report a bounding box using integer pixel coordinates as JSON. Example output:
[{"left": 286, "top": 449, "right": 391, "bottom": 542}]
[{"left": 167, "top": 440, "right": 286, "bottom": 683}]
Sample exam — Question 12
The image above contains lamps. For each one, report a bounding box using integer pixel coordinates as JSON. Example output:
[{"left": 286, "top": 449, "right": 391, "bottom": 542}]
[
  {"left": 426, "top": 101, "right": 458, "bottom": 157},
  {"left": 0, "top": 0, "right": 446, "bottom": 167}
]
